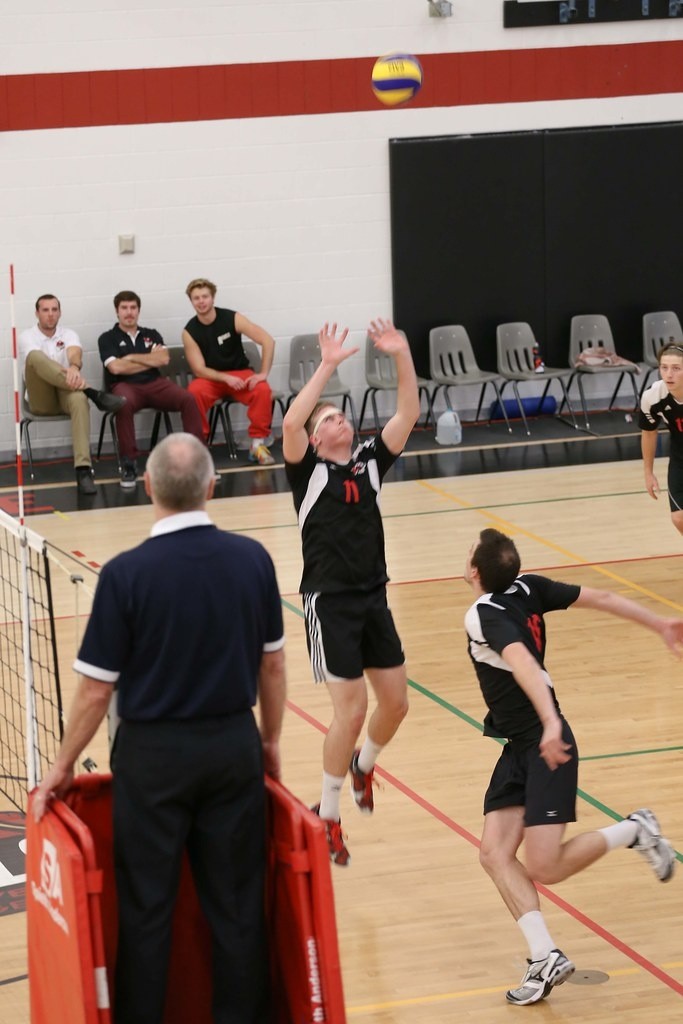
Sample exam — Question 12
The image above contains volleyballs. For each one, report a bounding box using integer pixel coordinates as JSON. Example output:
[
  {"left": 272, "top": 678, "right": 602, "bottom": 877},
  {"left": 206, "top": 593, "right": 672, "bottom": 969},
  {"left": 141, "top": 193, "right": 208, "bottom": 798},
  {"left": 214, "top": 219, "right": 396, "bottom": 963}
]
[{"left": 372, "top": 51, "right": 425, "bottom": 106}]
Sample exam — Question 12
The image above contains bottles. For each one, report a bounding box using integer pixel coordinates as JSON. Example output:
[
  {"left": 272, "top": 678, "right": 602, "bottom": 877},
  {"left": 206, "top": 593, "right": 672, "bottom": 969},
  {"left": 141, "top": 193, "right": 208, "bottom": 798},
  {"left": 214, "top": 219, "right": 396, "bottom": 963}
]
[
  {"left": 532, "top": 342, "right": 545, "bottom": 373},
  {"left": 234, "top": 432, "right": 278, "bottom": 450},
  {"left": 434, "top": 408, "right": 461, "bottom": 445}
]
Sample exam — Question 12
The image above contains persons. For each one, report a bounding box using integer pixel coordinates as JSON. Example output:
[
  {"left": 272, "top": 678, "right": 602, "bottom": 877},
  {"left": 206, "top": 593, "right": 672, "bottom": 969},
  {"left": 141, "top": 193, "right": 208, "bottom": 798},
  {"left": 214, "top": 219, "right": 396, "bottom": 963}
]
[
  {"left": 463, "top": 528, "right": 683, "bottom": 1005},
  {"left": 20, "top": 294, "right": 127, "bottom": 495},
  {"left": 282, "top": 318, "right": 422, "bottom": 865},
  {"left": 97, "top": 290, "right": 221, "bottom": 487},
  {"left": 31, "top": 434, "right": 286, "bottom": 1024},
  {"left": 638, "top": 341, "right": 683, "bottom": 534},
  {"left": 181, "top": 278, "right": 276, "bottom": 465}
]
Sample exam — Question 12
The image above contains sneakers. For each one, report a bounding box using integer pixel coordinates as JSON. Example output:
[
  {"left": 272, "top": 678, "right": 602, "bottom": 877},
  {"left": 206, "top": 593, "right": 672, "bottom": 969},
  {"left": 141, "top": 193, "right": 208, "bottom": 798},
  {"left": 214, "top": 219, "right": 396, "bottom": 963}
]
[
  {"left": 307, "top": 802, "right": 351, "bottom": 867},
  {"left": 120, "top": 457, "right": 136, "bottom": 488},
  {"left": 625, "top": 809, "right": 673, "bottom": 883},
  {"left": 349, "top": 748, "right": 375, "bottom": 813},
  {"left": 248, "top": 445, "right": 275, "bottom": 465},
  {"left": 505, "top": 949, "right": 575, "bottom": 1006}
]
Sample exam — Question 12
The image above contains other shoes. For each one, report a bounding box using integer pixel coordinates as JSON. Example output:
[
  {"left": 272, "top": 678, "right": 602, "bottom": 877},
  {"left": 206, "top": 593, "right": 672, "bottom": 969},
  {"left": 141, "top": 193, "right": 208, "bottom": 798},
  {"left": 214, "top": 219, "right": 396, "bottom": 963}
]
[
  {"left": 76, "top": 467, "right": 97, "bottom": 494},
  {"left": 95, "top": 391, "right": 126, "bottom": 410}
]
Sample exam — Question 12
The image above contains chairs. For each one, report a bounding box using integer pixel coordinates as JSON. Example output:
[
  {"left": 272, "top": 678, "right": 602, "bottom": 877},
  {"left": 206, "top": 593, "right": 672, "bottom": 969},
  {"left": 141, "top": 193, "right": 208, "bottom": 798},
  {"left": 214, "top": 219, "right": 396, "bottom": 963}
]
[
  {"left": 557, "top": 313, "right": 641, "bottom": 432},
  {"left": 284, "top": 334, "right": 362, "bottom": 445},
  {"left": 422, "top": 326, "right": 512, "bottom": 439},
  {"left": 487, "top": 321, "right": 578, "bottom": 436},
  {"left": 359, "top": 329, "right": 436, "bottom": 437},
  {"left": 634, "top": 311, "right": 683, "bottom": 413},
  {"left": 18, "top": 342, "right": 285, "bottom": 481}
]
